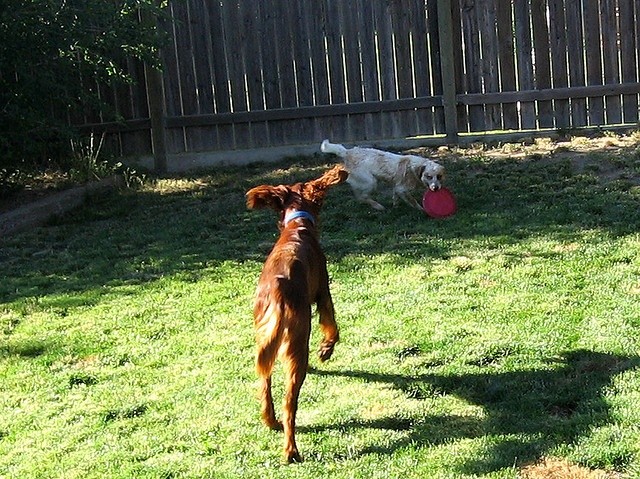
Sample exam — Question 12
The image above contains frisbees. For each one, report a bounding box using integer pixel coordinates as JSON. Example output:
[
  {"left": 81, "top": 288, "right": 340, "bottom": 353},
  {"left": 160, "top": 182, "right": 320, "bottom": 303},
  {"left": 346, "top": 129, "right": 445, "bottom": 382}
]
[{"left": 424, "top": 188, "right": 456, "bottom": 217}]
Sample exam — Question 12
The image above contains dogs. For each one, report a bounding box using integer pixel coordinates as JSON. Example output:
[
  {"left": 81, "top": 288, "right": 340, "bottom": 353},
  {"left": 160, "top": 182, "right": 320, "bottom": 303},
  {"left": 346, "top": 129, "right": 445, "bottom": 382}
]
[
  {"left": 246, "top": 165, "right": 349, "bottom": 464},
  {"left": 320, "top": 139, "right": 445, "bottom": 212}
]
